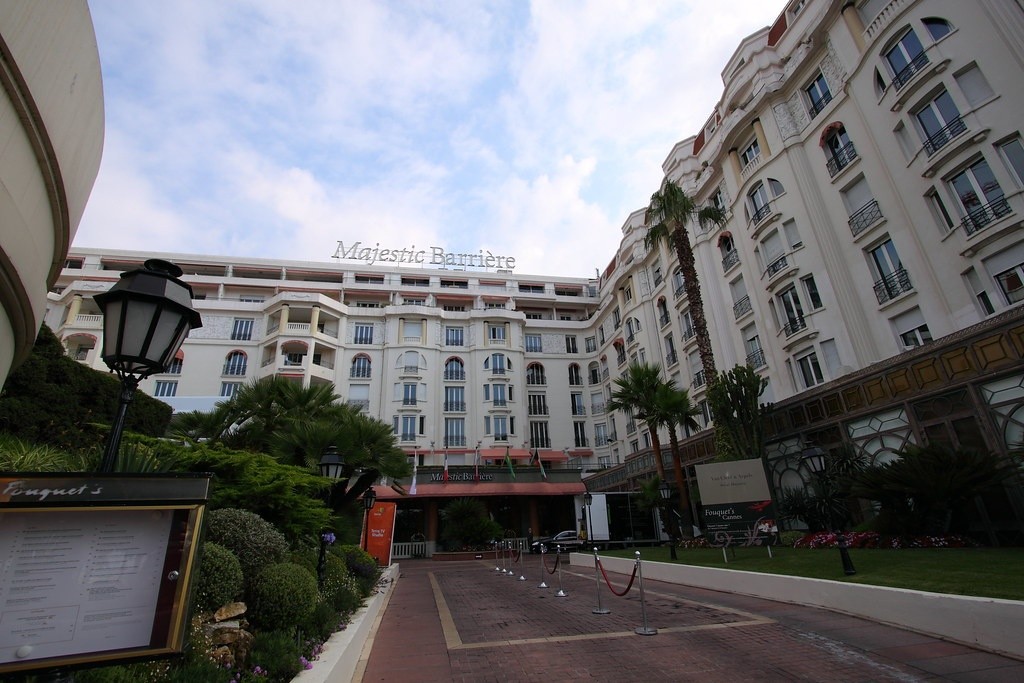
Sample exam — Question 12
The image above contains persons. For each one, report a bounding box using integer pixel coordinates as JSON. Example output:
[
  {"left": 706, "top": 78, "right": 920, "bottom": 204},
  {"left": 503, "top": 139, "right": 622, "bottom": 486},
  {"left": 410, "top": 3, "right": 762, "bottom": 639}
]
[{"left": 527, "top": 527, "right": 534, "bottom": 554}]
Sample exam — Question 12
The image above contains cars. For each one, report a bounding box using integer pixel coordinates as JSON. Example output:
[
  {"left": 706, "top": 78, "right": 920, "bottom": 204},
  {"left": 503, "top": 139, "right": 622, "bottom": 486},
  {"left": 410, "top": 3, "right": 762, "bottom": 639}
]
[{"left": 529, "top": 530, "right": 591, "bottom": 554}]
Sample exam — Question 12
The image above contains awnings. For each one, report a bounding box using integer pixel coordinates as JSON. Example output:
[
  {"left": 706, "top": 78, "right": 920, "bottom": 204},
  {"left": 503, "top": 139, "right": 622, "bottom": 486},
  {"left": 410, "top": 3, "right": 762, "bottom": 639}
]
[{"left": 480, "top": 448, "right": 593, "bottom": 475}]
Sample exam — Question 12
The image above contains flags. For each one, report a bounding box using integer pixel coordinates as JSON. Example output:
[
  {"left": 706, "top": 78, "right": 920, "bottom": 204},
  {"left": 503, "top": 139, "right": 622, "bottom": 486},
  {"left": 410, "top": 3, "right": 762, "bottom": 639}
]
[
  {"left": 474, "top": 449, "right": 480, "bottom": 485},
  {"left": 530, "top": 450, "right": 548, "bottom": 482},
  {"left": 443, "top": 449, "right": 448, "bottom": 487},
  {"left": 503, "top": 452, "right": 516, "bottom": 481},
  {"left": 409, "top": 461, "right": 417, "bottom": 494}
]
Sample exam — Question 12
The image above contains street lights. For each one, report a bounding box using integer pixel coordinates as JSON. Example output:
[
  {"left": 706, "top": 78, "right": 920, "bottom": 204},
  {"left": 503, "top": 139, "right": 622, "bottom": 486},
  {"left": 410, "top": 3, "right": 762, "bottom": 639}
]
[
  {"left": 800, "top": 440, "right": 857, "bottom": 575},
  {"left": 657, "top": 478, "right": 678, "bottom": 560},
  {"left": 583, "top": 491, "right": 594, "bottom": 552},
  {"left": 87, "top": 256, "right": 202, "bottom": 474},
  {"left": 316, "top": 444, "right": 345, "bottom": 594}
]
